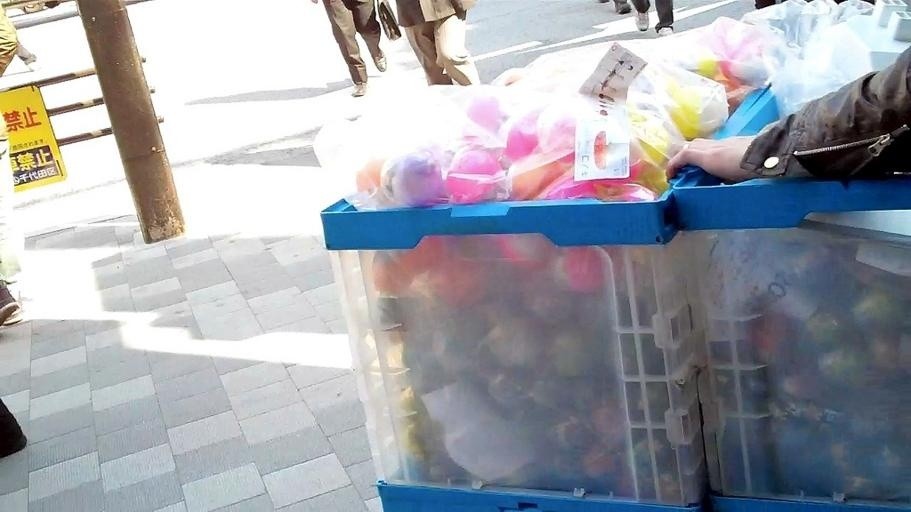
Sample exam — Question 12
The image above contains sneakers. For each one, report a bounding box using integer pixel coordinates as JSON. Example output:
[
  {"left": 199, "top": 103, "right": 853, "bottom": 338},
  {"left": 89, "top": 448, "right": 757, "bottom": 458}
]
[
  {"left": 636, "top": 10, "right": 649, "bottom": 31},
  {"left": 615, "top": 1, "right": 631, "bottom": 13},
  {"left": 658, "top": 27, "right": 672, "bottom": 36},
  {"left": 0, "top": 279, "right": 22, "bottom": 325}
]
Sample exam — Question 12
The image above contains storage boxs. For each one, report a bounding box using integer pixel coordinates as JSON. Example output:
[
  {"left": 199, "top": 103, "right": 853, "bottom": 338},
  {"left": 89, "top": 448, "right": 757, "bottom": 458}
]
[
  {"left": 670, "top": 72, "right": 911, "bottom": 512},
  {"left": 320, "top": 72, "right": 708, "bottom": 510}
]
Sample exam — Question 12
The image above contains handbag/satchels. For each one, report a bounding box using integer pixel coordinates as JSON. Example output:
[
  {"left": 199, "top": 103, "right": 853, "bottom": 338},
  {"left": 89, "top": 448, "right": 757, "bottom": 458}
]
[{"left": 378, "top": 0, "right": 401, "bottom": 40}]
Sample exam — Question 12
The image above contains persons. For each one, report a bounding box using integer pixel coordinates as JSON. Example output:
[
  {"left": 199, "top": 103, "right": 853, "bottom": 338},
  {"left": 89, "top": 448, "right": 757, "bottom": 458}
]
[
  {"left": 1, "top": 2, "right": 37, "bottom": 326},
  {"left": 629, "top": 1, "right": 675, "bottom": 38},
  {"left": 395, "top": 0, "right": 479, "bottom": 86},
  {"left": 662, "top": 46, "right": 911, "bottom": 182},
  {"left": 310, "top": 1, "right": 387, "bottom": 97},
  {"left": 597, "top": 1, "right": 631, "bottom": 14}
]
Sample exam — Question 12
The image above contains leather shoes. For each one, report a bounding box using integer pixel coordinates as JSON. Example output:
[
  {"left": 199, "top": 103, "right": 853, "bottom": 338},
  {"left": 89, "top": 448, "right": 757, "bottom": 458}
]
[
  {"left": 373, "top": 48, "right": 386, "bottom": 72},
  {"left": 353, "top": 83, "right": 366, "bottom": 96}
]
